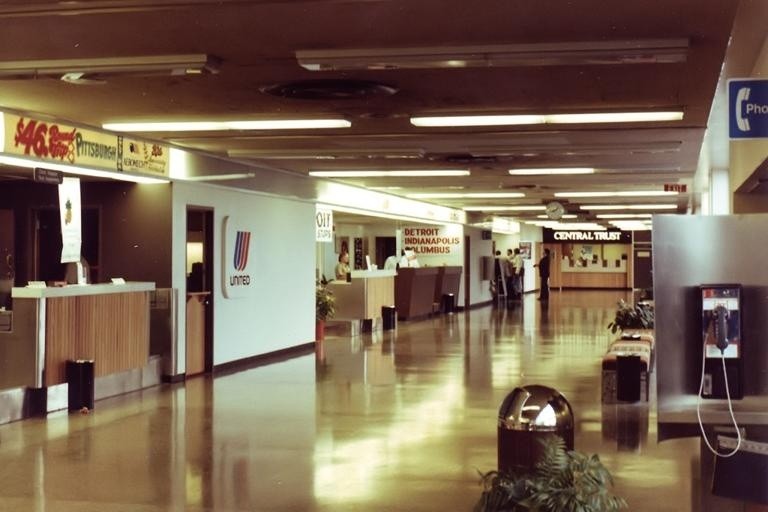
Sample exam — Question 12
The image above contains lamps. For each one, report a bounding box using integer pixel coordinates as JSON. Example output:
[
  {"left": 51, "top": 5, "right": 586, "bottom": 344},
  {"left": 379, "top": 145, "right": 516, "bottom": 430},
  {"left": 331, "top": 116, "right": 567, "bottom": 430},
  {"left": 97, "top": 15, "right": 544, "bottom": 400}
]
[
  {"left": 296, "top": 36, "right": 690, "bottom": 70},
  {"left": 1, "top": 54, "right": 222, "bottom": 79}
]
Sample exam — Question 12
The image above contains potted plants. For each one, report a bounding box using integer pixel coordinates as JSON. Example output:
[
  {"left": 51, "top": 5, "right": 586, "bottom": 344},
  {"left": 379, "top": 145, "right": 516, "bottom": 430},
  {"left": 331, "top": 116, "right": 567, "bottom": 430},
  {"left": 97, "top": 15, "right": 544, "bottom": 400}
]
[{"left": 316, "top": 286, "right": 337, "bottom": 341}]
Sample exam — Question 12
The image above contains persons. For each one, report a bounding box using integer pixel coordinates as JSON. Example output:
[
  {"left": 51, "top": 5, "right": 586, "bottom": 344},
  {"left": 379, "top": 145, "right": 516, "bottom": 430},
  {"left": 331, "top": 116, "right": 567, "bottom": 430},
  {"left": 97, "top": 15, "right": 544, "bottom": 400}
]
[
  {"left": 568, "top": 248, "right": 599, "bottom": 269},
  {"left": 334, "top": 253, "right": 351, "bottom": 279},
  {"left": 495, "top": 247, "right": 525, "bottom": 298},
  {"left": 399, "top": 246, "right": 418, "bottom": 268},
  {"left": 534, "top": 248, "right": 550, "bottom": 300}
]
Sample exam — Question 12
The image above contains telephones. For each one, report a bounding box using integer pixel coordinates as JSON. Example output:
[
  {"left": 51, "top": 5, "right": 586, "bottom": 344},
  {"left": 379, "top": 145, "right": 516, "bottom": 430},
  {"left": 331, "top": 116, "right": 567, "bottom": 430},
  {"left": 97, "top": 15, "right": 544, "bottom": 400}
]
[{"left": 715, "top": 307, "right": 728, "bottom": 350}]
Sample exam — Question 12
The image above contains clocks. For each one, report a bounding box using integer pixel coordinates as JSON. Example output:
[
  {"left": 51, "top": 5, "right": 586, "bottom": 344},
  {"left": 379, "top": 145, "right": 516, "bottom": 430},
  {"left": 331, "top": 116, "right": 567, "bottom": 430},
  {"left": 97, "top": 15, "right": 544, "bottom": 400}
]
[{"left": 546, "top": 202, "right": 564, "bottom": 220}]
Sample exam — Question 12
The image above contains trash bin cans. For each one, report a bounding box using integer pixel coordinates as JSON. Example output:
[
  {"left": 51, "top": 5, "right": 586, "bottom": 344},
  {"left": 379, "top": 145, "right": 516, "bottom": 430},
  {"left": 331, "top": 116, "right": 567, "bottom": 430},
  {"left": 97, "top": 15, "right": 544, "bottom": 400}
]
[
  {"left": 66, "top": 358, "right": 95, "bottom": 413},
  {"left": 444, "top": 294, "right": 454, "bottom": 313},
  {"left": 381, "top": 304, "right": 396, "bottom": 331},
  {"left": 497, "top": 385, "right": 574, "bottom": 478}
]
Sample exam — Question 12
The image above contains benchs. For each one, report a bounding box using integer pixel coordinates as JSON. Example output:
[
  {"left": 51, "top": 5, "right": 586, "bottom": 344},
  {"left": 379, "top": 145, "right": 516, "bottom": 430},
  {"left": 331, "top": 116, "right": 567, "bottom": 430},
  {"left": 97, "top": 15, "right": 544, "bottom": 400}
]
[{"left": 602, "top": 336, "right": 654, "bottom": 405}]
